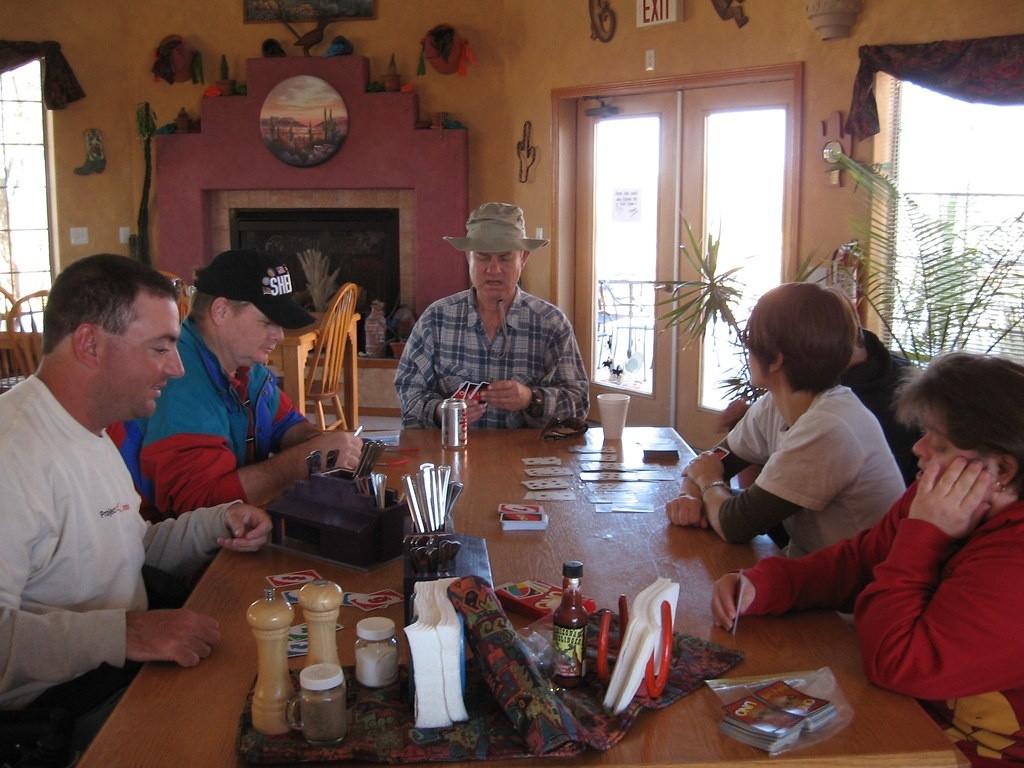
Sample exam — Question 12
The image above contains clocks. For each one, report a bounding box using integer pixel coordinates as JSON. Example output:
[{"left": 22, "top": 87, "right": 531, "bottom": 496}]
[{"left": 816, "top": 111, "right": 852, "bottom": 188}]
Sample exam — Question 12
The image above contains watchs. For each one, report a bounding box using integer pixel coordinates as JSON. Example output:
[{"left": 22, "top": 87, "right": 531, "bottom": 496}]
[{"left": 526, "top": 388, "right": 543, "bottom": 418}]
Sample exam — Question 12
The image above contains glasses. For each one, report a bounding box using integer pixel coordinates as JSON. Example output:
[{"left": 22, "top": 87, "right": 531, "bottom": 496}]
[
  {"left": 540, "top": 416, "right": 590, "bottom": 441},
  {"left": 737, "top": 330, "right": 756, "bottom": 352}
]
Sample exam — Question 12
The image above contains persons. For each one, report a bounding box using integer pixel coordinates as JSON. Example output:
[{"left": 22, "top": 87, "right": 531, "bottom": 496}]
[
  {"left": 663, "top": 282, "right": 907, "bottom": 621},
  {"left": 719, "top": 287, "right": 927, "bottom": 488},
  {"left": 392, "top": 202, "right": 591, "bottom": 428},
  {"left": 712, "top": 354, "right": 1024, "bottom": 768},
  {"left": 0, "top": 253, "right": 273, "bottom": 768},
  {"left": 103, "top": 247, "right": 362, "bottom": 611}
]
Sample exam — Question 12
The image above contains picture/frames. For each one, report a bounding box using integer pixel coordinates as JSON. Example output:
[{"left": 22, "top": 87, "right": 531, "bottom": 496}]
[{"left": 243, "top": 0, "right": 377, "bottom": 24}]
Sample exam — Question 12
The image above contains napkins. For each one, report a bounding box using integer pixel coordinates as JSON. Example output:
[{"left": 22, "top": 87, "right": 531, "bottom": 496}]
[
  {"left": 404, "top": 578, "right": 469, "bottom": 728},
  {"left": 601, "top": 577, "right": 679, "bottom": 715}
]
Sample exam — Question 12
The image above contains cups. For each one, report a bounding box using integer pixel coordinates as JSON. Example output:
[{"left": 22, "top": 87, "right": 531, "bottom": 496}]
[{"left": 597, "top": 394, "right": 631, "bottom": 439}]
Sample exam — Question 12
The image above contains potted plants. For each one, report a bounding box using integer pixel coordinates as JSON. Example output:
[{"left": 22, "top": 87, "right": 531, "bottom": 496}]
[
  {"left": 216, "top": 55, "right": 237, "bottom": 96},
  {"left": 174, "top": 107, "right": 192, "bottom": 130},
  {"left": 381, "top": 53, "right": 401, "bottom": 92},
  {"left": 363, "top": 296, "right": 407, "bottom": 370}
]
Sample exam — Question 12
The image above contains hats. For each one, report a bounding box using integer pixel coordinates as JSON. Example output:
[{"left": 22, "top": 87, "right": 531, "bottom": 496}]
[
  {"left": 443, "top": 202, "right": 549, "bottom": 253},
  {"left": 194, "top": 249, "right": 318, "bottom": 330}
]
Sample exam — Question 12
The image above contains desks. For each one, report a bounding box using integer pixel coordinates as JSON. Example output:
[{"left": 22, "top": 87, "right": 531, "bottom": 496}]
[
  {"left": 612, "top": 324, "right": 654, "bottom": 382},
  {"left": 73, "top": 427, "right": 974, "bottom": 768},
  {"left": 0, "top": 310, "right": 361, "bottom": 432}
]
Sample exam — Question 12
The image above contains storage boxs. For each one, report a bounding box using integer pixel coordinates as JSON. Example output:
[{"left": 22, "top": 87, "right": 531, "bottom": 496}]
[{"left": 494, "top": 577, "right": 598, "bottom": 619}]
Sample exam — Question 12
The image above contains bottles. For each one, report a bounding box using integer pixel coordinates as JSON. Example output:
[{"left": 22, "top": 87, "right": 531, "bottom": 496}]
[
  {"left": 297, "top": 579, "right": 345, "bottom": 686},
  {"left": 247, "top": 592, "right": 297, "bottom": 736},
  {"left": 355, "top": 617, "right": 399, "bottom": 687},
  {"left": 298, "top": 663, "right": 347, "bottom": 745},
  {"left": 552, "top": 561, "right": 588, "bottom": 686}
]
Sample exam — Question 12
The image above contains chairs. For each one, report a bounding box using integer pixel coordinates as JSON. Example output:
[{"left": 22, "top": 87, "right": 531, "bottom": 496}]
[
  {"left": 157, "top": 270, "right": 191, "bottom": 324},
  {"left": 274, "top": 284, "right": 358, "bottom": 431},
  {"left": 596, "top": 280, "right": 643, "bottom": 370},
  {"left": 0, "top": 286, "right": 51, "bottom": 394}
]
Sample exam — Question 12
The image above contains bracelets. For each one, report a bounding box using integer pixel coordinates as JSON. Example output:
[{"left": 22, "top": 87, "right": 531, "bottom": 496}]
[{"left": 698, "top": 481, "right": 729, "bottom": 500}]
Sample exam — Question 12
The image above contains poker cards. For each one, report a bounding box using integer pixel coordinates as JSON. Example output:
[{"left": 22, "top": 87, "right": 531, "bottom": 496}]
[
  {"left": 451, "top": 381, "right": 490, "bottom": 407},
  {"left": 353, "top": 425, "right": 364, "bottom": 436},
  {"left": 265, "top": 568, "right": 406, "bottom": 658},
  {"left": 497, "top": 503, "right": 549, "bottom": 532},
  {"left": 731, "top": 565, "right": 746, "bottom": 636},
  {"left": 521, "top": 442, "right": 682, "bottom": 514},
  {"left": 713, "top": 445, "right": 731, "bottom": 460}
]
